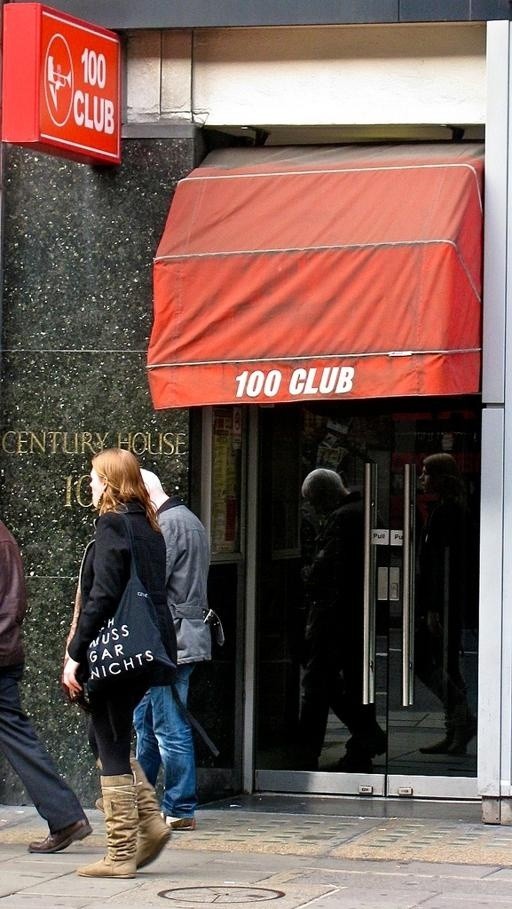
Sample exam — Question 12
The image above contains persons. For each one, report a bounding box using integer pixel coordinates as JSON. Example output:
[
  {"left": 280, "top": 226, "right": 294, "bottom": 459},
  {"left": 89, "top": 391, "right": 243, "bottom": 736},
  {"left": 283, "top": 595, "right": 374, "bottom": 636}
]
[
  {"left": 59, "top": 447, "right": 182, "bottom": 879},
  {"left": 397, "top": 451, "right": 476, "bottom": 755},
  {"left": 87, "top": 466, "right": 213, "bottom": 830},
  {"left": 286, "top": 463, "right": 385, "bottom": 775},
  {"left": 2, "top": 517, "right": 95, "bottom": 854}
]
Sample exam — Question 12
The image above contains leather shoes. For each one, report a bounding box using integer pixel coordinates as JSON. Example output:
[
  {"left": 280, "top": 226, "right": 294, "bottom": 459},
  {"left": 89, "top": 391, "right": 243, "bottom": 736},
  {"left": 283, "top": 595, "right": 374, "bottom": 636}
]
[{"left": 27, "top": 815, "right": 93, "bottom": 853}]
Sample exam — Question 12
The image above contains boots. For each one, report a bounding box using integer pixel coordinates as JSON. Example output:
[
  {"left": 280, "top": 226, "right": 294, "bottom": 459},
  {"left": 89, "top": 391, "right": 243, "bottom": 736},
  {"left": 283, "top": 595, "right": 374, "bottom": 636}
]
[
  {"left": 420, "top": 703, "right": 476, "bottom": 756},
  {"left": 77, "top": 750, "right": 172, "bottom": 879}
]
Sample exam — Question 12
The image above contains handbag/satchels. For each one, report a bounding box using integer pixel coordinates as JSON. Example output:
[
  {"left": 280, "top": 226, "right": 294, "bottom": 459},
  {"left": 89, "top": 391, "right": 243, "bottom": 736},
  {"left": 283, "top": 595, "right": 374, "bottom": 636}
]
[{"left": 86, "top": 575, "right": 179, "bottom": 698}]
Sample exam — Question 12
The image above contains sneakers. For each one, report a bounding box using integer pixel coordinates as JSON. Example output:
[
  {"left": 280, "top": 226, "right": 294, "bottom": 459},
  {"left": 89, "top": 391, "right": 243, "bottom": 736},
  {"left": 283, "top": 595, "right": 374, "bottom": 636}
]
[
  {"left": 336, "top": 722, "right": 388, "bottom": 772},
  {"left": 160, "top": 813, "right": 196, "bottom": 831}
]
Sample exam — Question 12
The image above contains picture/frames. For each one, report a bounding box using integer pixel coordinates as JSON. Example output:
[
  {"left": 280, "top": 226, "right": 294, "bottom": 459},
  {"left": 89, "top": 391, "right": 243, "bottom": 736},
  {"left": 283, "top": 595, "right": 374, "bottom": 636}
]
[{"left": 200, "top": 405, "right": 248, "bottom": 565}]
